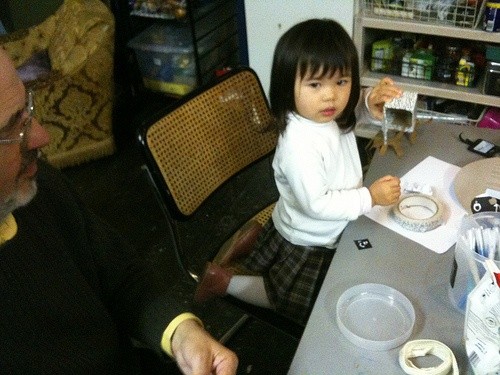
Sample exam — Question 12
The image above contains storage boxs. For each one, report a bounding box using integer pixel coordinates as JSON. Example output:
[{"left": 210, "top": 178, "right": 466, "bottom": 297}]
[
  {"left": 126, "top": 21, "right": 238, "bottom": 96},
  {"left": 401, "top": 52, "right": 436, "bottom": 80},
  {"left": 383, "top": 90, "right": 418, "bottom": 133}
]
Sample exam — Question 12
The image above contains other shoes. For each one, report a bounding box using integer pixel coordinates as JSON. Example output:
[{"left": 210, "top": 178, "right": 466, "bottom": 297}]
[
  {"left": 216, "top": 220, "right": 263, "bottom": 265},
  {"left": 195, "top": 262, "right": 232, "bottom": 303}
]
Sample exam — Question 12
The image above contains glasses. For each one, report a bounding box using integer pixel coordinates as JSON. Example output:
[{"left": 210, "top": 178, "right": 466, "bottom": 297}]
[{"left": 0, "top": 89, "right": 37, "bottom": 153}]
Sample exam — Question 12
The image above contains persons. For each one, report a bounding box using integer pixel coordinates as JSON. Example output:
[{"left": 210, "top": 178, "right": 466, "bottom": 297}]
[
  {"left": 193, "top": 18, "right": 401, "bottom": 327},
  {"left": 0, "top": 41, "right": 239, "bottom": 375}
]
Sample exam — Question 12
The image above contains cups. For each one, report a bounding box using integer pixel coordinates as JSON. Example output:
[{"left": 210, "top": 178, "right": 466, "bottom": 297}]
[{"left": 448, "top": 211, "right": 500, "bottom": 316}]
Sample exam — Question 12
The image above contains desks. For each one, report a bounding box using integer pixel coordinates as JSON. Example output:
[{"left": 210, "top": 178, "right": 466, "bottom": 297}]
[{"left": 288, "top": 121, "right": 500, "bottom": 375}]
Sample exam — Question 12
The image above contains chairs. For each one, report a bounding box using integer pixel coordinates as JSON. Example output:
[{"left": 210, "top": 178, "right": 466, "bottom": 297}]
[{"left": 134, "top": 66, "right": 305, "bottom": 347}]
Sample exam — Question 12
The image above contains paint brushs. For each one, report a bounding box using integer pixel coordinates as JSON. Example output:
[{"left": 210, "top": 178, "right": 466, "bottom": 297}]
[{"left": 455, "top": 224, "right": 500, "bottom": 312}]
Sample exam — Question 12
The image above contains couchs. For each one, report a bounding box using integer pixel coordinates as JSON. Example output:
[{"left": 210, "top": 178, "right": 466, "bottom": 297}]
[{"left": 0, "top": 0, "right": 115, "bottom": 169}]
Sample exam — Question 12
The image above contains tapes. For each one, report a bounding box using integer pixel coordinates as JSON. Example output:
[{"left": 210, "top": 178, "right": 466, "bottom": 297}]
[{"left": 391, "top": 193, "right": 444, "bottom": 233}]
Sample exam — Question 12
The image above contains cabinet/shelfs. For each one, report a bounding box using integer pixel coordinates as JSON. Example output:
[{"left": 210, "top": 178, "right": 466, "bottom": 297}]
[
  {"left": 353, "top": 16, "right": 500, "bottom": 139},
  {"left": 128, "top": 0, "right": 241, "bottom": 86}
]
